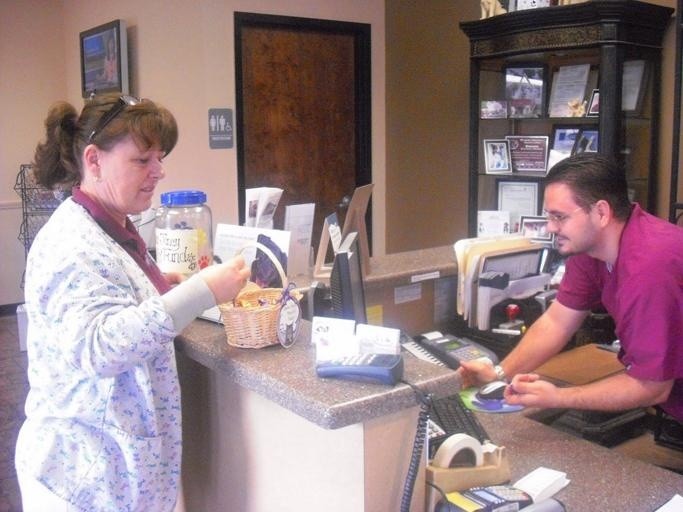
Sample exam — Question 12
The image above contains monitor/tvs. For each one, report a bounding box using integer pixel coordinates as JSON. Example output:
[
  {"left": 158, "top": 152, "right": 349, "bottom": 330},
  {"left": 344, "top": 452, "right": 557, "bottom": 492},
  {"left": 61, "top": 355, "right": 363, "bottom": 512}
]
[
  {"left": 330, "top": 232, "right": 367, "bottom": 330},
  {"left": 79, "top": 19, "right": 122, "bottom": 98}
]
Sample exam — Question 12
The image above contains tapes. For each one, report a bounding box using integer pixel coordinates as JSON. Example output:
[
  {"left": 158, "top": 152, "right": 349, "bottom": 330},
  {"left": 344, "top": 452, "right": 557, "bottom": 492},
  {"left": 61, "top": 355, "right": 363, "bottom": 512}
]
[{"left": 431, "top": 431, "right": 499, "bottom": 470}]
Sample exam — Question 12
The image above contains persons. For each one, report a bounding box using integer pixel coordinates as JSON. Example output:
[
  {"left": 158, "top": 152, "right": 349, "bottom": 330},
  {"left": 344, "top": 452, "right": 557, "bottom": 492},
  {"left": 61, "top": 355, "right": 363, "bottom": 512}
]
[
  {"left": 453, "top": 151, "right": 683, "bottom": 435},
  {"left": 15, "top": 93, "right": 251, "bottom": 512}
]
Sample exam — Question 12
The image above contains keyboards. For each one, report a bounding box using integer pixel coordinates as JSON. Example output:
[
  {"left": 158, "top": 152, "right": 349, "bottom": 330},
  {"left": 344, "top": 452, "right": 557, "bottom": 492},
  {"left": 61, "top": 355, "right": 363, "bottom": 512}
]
[{"left": 427, "top": 394, "right": 492, "bottom": 464}]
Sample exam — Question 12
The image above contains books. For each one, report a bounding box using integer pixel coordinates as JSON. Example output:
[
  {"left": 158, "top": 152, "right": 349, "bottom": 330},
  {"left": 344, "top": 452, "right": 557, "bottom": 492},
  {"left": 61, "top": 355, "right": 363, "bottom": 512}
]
[{"left": 455, "top": 232, "right": 545, "bottom": 331}]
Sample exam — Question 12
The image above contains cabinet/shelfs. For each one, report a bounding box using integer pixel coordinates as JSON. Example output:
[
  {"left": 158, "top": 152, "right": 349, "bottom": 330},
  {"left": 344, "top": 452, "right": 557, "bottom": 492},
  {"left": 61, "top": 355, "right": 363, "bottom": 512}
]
[{"left": 459, "top": 3, "right": 676, "bottom": 240}]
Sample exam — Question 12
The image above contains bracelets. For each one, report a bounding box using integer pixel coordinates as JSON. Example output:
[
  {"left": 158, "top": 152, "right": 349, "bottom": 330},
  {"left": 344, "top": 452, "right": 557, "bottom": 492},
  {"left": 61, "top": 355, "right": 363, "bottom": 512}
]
[{"left": 493, "top": 364, "right": 505, "bottom": 381}]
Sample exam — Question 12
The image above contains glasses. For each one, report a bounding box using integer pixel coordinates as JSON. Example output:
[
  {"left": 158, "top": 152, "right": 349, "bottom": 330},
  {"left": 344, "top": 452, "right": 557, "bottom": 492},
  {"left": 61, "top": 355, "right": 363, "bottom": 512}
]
[
  {"left": 87, "top": 94, "right": 152, "bottom": 144},
  {"left": 543, "top": 207, "right": 583, "bottom": 226}
]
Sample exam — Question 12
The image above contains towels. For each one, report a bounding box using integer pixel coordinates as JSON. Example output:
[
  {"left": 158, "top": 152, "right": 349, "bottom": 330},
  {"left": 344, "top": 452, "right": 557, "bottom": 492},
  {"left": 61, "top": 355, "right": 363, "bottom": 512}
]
[{"left": 512, "top": 466, "right": 571, "bottom": 503}]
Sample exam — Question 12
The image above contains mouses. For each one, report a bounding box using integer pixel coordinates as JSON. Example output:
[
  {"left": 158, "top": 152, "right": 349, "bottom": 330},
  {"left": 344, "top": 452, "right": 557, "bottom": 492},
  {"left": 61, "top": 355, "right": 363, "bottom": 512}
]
[{"left": 479, "top": 381, "right": 509, "bottom": 399}]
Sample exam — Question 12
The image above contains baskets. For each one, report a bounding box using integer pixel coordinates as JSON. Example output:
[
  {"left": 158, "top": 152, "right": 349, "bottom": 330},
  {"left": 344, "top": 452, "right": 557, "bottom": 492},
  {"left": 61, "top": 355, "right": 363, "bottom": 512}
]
[{"left": 218, "top": 242, "right": 304, "bottom": 349}]
